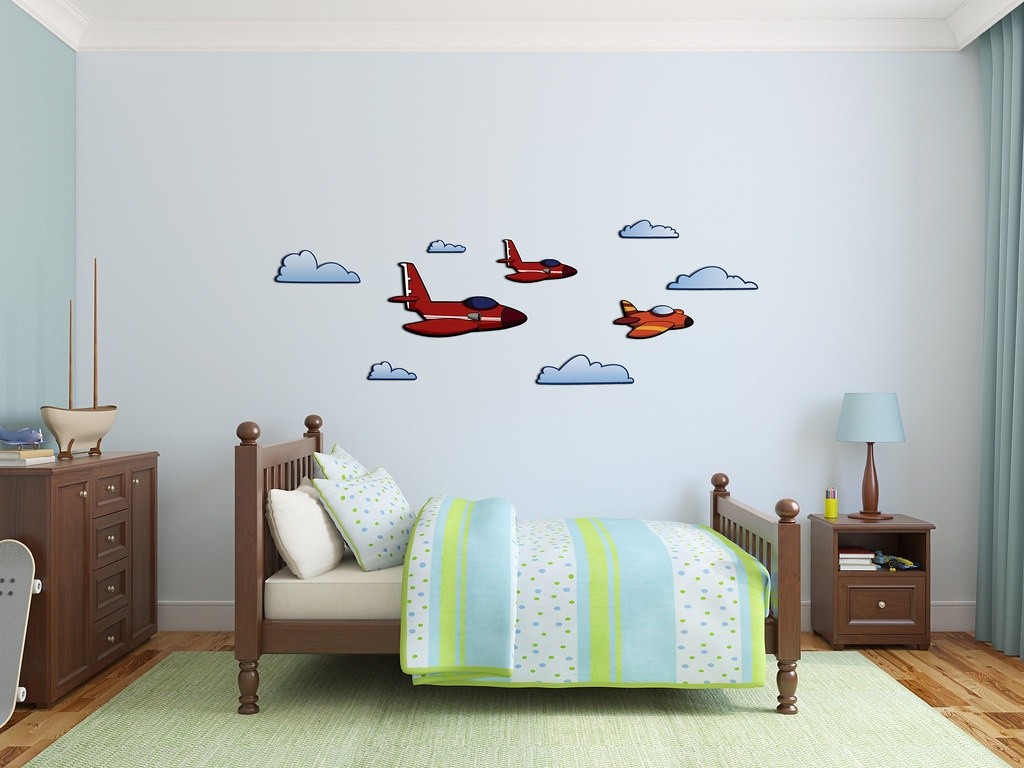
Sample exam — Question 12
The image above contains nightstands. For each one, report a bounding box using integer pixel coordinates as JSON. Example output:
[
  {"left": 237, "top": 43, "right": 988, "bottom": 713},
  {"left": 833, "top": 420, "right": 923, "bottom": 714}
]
[{"left": 807, "top": 513, "right": 936, "bottom": 651}]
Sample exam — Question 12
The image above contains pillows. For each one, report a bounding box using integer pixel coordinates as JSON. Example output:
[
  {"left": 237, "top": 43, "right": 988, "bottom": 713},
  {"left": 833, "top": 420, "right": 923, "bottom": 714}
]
[
  {"left": 311, "top": 465, "right": 417, "bottom": 571},
  {"left": 312, "top": 442, "right": 372, "bottom": 480},
  {"left": 265, "top": 476, "right": 345, "bottom": 579}
]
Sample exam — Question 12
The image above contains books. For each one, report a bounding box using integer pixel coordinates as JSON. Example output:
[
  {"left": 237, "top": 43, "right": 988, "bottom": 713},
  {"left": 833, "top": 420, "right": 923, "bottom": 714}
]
[
  {"left": 839, "top": 546, "right": 877, "bottom": 570},
  {"left": 0, "top": 449, "right": 55, "bottom": 466}
]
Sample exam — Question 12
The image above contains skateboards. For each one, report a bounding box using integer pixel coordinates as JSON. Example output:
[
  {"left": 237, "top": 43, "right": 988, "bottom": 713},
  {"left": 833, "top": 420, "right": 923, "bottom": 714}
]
[{"left": 0, "top": 538, "right": 43, "bottom": 730}]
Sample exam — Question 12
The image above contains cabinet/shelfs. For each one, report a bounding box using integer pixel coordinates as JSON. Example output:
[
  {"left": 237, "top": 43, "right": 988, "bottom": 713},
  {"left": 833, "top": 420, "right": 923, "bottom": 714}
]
[{"left": 0, "top": 449, "right": 161, "bottom": 709}]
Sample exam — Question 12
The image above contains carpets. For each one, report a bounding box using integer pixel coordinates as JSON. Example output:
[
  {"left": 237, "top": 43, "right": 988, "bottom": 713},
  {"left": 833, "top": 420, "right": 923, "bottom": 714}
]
[{"left": 23, "top": 647, "right": 1005, "bottom": 767}]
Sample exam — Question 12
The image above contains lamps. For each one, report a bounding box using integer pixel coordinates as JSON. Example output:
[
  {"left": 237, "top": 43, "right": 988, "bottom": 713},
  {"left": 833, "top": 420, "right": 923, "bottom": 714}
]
[{"left": 835, "top": 392, "right": 906, "bottom": 520}]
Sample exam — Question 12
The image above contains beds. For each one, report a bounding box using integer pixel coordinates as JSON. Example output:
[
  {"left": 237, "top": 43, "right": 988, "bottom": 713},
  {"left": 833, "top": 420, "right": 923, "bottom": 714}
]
[{"left": 234, "top": 414, "right": 802, "bottom": 715}]
